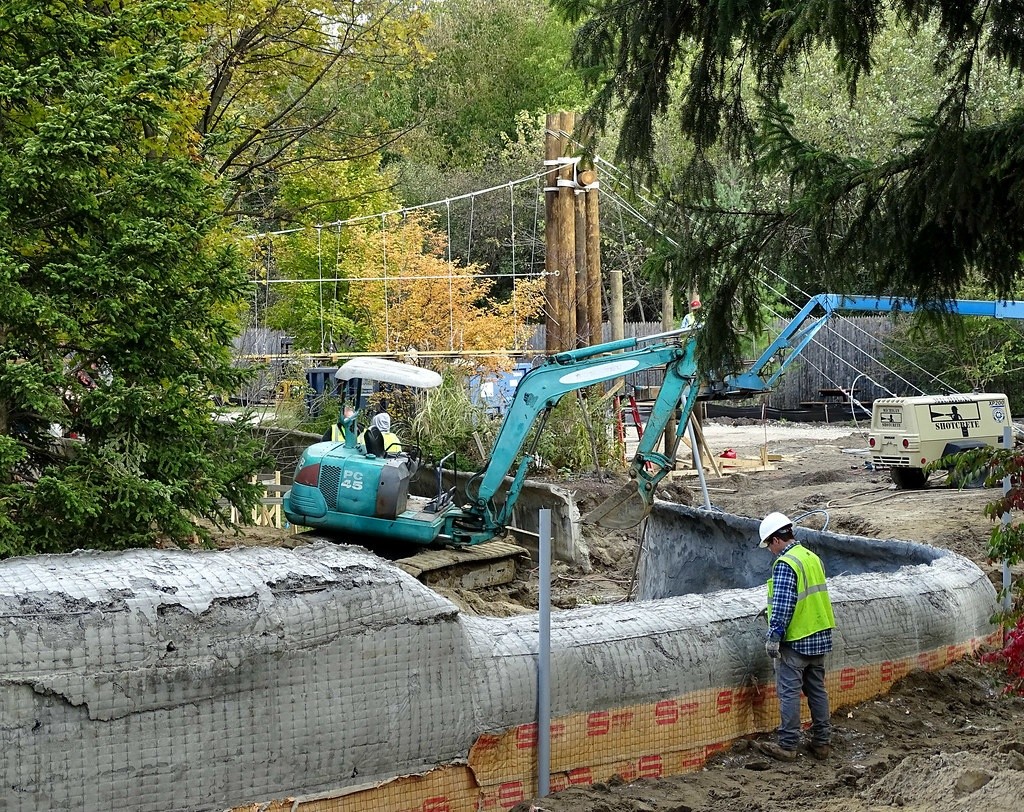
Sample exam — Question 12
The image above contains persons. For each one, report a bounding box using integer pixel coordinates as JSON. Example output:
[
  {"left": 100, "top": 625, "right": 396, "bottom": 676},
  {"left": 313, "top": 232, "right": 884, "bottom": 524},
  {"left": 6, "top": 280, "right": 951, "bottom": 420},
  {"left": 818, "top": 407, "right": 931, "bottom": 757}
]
[
  {"left": 681, "top": 300, "right": 706, "bottom": 328},
  {"left": 758, "top": 512, "right": 836, "bottom": 761},
  {"left": 320, "top": 406, "right": 366, "bottom": 442},
  {"left": 357, "top": 413, "right": 402, "bottom": 452}
]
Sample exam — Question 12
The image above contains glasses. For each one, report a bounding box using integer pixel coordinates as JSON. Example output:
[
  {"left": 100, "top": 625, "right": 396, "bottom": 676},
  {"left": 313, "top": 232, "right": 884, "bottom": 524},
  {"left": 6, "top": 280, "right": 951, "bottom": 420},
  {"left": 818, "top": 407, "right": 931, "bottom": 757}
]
[{"left": 766, "top": 537, "right": 778, "bottom": 551}]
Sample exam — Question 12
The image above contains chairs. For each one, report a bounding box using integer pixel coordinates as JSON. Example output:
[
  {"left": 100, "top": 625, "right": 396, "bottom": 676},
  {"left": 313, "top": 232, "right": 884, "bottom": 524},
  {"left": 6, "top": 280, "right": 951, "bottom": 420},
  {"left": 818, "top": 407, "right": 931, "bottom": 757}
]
[{"left": 366, "top": 426, "right": 410, "bottom": 465}]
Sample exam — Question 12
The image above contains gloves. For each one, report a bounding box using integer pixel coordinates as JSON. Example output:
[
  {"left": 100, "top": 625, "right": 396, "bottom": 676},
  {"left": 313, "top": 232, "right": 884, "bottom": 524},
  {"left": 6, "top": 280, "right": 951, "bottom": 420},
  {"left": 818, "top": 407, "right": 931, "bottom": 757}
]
[{"left": 765, "top": 641, "right": 781, "bottom": 658}]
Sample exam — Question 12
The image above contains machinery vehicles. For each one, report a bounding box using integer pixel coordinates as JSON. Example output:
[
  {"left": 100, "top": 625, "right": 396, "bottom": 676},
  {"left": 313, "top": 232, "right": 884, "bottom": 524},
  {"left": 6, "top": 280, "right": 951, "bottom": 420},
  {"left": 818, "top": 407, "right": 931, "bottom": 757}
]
[{"left": 280, "top": 318, "right": 713, "bottom": 598}]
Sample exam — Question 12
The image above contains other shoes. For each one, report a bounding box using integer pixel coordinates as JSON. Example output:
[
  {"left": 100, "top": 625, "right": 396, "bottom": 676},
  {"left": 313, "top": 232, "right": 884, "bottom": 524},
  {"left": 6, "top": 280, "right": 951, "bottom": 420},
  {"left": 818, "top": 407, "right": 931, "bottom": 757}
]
[
  {"left": 806, "top": 741, "right": 829, "bottom": 760},
  {"left": 762, "top": 741, "right": 796, "bottom": 761}
]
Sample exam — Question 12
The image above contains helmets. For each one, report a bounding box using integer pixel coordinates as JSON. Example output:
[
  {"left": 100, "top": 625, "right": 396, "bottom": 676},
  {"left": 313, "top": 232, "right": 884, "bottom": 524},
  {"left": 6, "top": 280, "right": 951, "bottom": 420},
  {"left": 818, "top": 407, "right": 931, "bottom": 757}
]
[{"left": 759, "top": 512, "right": 796, "bottom": 548}]
